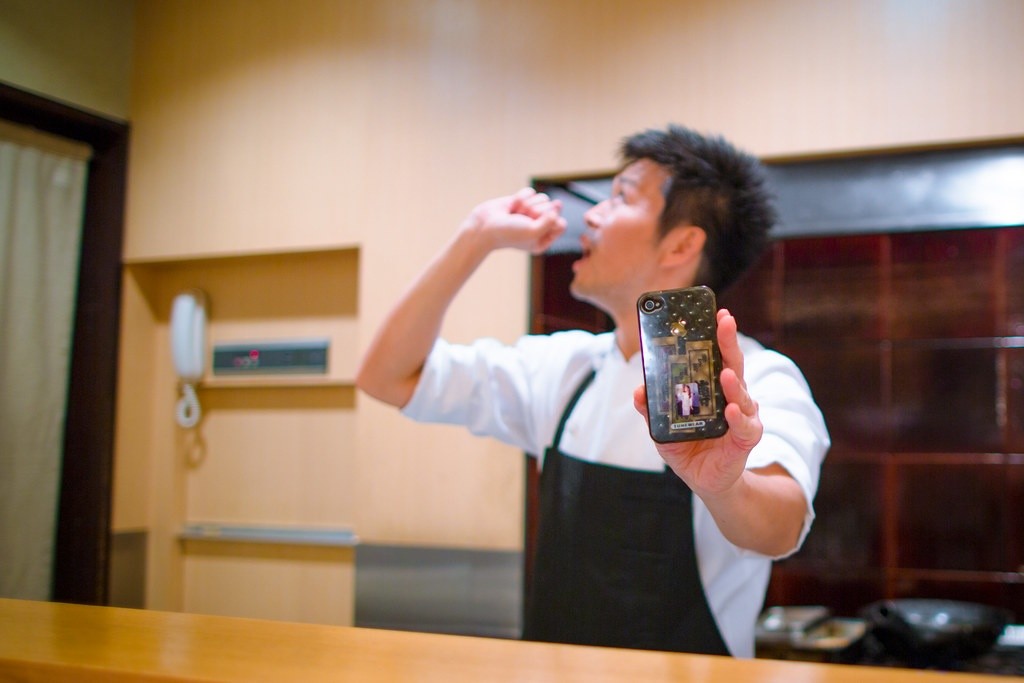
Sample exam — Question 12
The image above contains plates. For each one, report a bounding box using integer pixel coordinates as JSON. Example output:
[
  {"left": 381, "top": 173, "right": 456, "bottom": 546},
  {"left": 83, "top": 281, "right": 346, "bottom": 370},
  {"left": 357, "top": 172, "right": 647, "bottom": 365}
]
[{"left": 755, "top": 605, "right": 869, "bottom": 650}]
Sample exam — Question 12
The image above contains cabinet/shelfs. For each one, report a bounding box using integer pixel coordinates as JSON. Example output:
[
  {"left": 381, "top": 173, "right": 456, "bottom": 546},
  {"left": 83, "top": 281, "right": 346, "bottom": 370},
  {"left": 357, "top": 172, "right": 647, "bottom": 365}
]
[{"left": 523, "top": 135, "right": 1024, "bottom": 654}]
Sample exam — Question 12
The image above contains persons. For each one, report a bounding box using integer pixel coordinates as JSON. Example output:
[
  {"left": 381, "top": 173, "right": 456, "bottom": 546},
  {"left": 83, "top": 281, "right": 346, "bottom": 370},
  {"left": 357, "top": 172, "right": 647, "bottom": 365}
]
[
  {"left": 677, "top": 385, "right": 694, "bottom": 420},
  {"left": 354, "top": 124, "right": 832, "bottom": 659}
]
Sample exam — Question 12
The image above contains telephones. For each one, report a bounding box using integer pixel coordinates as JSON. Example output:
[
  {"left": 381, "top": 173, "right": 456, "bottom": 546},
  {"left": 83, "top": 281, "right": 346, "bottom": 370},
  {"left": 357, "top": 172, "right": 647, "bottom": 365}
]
[{"left": 170, "top": 287, "right": 208, "bottom": 382}]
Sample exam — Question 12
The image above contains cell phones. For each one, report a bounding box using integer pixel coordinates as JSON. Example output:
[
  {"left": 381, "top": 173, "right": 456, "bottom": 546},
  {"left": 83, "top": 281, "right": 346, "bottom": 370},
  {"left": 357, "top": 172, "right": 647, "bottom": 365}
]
[{"left": 637, "top": 287, "right": 729, "bottom": 444}]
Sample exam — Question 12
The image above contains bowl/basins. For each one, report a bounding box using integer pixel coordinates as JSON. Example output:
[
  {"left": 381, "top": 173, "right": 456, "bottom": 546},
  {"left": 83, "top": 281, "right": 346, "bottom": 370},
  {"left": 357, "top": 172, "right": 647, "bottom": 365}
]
[{"left": 869, "top": 597, "right": 1006, "bottom": 671}]
